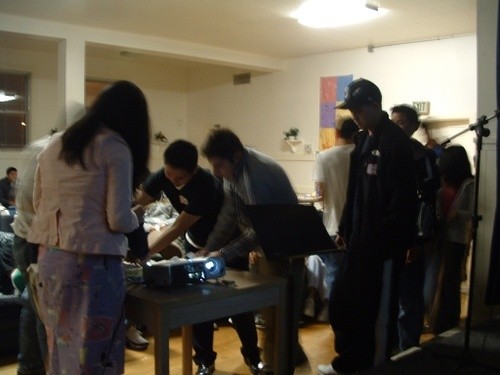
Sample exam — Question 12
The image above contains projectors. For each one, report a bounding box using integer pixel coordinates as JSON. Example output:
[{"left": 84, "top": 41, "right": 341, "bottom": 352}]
[{"left": 143, "top": 256, "right": 226, "bottom": 289}]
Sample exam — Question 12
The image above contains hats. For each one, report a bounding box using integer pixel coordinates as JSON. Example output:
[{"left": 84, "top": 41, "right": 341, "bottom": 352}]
[{"left": 333, "top": 77, "right": 382, "bottom": 110}]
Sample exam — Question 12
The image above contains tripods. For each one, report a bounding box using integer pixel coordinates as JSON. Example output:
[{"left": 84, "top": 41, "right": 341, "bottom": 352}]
[{"left": 433, "top": 112, "right": 500, "bottom": 375}]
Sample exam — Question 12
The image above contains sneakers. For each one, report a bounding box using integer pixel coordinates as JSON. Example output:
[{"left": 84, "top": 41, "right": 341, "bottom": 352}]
[{"left": 125, "top": 325, "right": 149, "bottom": 350}]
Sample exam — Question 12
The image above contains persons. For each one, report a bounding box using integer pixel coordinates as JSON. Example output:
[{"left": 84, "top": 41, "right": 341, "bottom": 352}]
[
  {"left": 124, "top": 225, "right": 155, "bottom": 350},
  {"left": 9, "top": 129, "right": 57, "bottom": 375},
  {"left": 297, "top": 117, "right": 360, "bottom": 300},
  {"left": 317, "top": 78, "right": 420, "bottom": 375},
  {"left": 391, "top": 104, "right": 476, "bottom": 350},
  {"left": 134, "top": 139, "right": 265, "bottom": 375},
  {"left": 0, "top": 167, "right": 19, "bottom": 208},
  {"left": 26, "top": 80, "right": 151, "bottom": 375},
  {"left": 194, "top": 130, "right": 309, "bottom": 375}
]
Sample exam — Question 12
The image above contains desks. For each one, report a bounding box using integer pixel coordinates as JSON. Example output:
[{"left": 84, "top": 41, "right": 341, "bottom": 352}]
[{"left": 124, "top": 270, "right": 288, "bottom": 375}]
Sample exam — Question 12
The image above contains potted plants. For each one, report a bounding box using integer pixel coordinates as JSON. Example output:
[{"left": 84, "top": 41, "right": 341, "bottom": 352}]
[{"left": 284, "top": 128, "right": 299, "bottom": 140}]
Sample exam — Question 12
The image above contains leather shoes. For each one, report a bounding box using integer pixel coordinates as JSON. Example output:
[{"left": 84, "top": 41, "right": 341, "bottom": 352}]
[
  {"left": 196, "top": 361, "right": 215, "bottom": 375},
  {"left": 244, "top": 356, "right": 263, "bottom": 375}
]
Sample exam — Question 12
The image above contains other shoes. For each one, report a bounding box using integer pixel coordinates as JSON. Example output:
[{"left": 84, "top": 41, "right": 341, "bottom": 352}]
[{"left": 317, "top": 364, "right": 337, "bottom": 374}]
